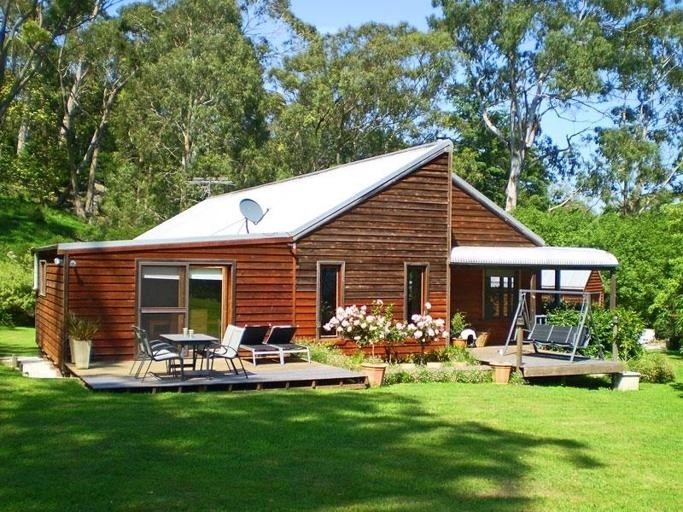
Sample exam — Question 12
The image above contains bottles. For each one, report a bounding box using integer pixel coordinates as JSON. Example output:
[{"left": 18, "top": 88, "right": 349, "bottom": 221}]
[{"left": 183, "top": 328, "right": 194, "bottom": 339}]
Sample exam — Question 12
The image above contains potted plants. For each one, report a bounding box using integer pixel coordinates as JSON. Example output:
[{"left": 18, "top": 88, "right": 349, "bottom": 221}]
[
  {"left": 452, "top": 312, "right": 467, "bottom": 349},
  {"left": 66, "top": 309, "right": 104, "bottom": 369},
  {"left": 361, "top": 358, "right": 387, "bottom": 388}
]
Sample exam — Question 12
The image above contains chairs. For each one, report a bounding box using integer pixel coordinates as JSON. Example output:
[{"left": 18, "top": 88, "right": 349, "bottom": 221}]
[{"left": 129, "top": 323, "right": 310, "bottom": 382}]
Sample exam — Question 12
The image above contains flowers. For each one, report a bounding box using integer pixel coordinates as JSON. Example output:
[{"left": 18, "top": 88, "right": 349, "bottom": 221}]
[{"left": 323, "top": 299, "right": 451, "bottom": 348}]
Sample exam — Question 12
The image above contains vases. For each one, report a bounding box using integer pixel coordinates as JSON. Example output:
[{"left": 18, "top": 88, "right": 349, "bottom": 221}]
[{"left": 489, "top": 360, "right": 512, "bottom": 384}]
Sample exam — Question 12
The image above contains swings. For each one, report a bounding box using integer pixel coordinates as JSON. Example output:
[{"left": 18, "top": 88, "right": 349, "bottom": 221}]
[{"left": 522, "top": 296, "right": 591, "bottom": 348}]
